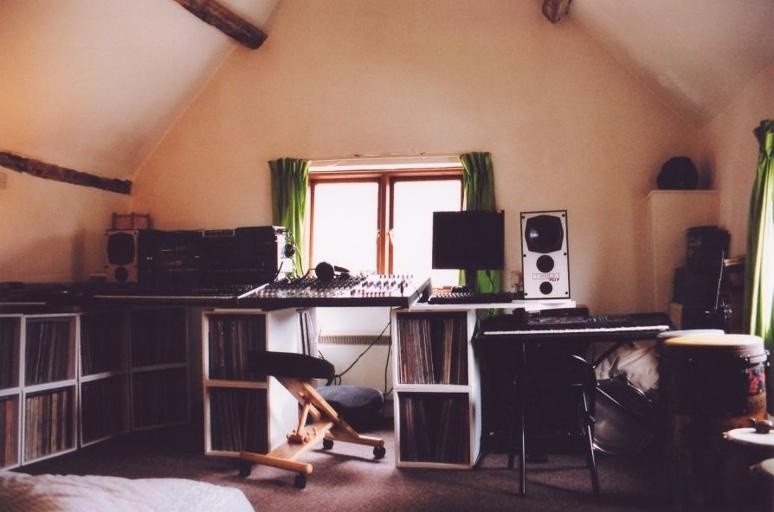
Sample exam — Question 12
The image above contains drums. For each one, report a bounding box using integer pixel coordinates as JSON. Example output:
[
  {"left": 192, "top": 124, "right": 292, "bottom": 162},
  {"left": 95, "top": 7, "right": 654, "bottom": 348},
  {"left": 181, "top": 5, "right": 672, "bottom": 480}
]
[{"left": 656, "top": 328, "right": 768, "bottom": 430}]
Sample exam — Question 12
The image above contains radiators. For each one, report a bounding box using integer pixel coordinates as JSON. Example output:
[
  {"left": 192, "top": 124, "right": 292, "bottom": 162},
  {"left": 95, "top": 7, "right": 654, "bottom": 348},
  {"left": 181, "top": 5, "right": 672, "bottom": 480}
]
[{"left": 315, "top": 331, "right": 393, "bottom": 400}]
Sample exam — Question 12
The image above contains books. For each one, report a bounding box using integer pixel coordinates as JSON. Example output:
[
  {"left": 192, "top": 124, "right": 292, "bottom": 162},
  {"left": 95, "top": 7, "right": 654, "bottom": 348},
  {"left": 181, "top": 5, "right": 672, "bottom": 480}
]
[
  {"left": 208, "top": 315, "right": 269, "bottom": 455},
  {"left": 0, "top": 309, "right": 193, "bottom": 471}
]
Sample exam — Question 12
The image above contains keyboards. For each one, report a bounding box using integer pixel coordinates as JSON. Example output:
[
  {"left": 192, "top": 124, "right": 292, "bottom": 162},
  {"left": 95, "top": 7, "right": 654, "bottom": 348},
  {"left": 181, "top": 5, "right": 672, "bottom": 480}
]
[{"left": 427, "top": 292, "right": 512, "bottom": 303}]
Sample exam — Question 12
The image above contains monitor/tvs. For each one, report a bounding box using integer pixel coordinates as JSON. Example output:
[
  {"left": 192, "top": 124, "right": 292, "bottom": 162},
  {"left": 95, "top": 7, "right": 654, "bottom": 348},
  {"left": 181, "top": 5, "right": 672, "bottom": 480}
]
[{"left": 431, "top": 210, "right": 505, "bottom": 294}]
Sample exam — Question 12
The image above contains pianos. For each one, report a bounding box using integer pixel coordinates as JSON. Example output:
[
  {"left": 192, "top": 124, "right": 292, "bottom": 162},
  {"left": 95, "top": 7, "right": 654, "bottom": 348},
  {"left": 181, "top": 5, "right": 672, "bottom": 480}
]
[{"left": 474, "top": 311, "right": 673, "bottom": 340}]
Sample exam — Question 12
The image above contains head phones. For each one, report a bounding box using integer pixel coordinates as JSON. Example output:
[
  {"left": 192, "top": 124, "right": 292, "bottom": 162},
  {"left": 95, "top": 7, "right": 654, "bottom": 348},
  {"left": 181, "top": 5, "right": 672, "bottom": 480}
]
[{"left": 314, "top": 263, "right": 349, "bottom": 281}]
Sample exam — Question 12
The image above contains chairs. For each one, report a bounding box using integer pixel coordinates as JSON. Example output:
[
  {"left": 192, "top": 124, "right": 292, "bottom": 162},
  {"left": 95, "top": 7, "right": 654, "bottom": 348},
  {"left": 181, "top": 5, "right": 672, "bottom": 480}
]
[
  {"left": 503, "top": 353, "right": 604, "bottom": 497},
  {"left": 242, "top": 349, "right": 384, "bottom": 478}
]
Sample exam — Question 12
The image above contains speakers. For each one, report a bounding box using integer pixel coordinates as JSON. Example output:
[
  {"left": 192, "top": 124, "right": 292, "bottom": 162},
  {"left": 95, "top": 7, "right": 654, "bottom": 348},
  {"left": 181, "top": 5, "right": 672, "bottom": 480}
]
[
  {"left": 106, "top": 229, "right": 138, "bottom": 283},
  {"left": 519, "top": 209, "right": 570, "bottom": 299}
]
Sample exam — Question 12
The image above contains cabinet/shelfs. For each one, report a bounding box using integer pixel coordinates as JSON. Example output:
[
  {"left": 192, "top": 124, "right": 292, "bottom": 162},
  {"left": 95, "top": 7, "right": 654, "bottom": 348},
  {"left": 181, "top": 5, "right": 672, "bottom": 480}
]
[
  {"left": 130, "top": 302, "right": 191, "bottom": 433},
  {"left": 202, "top": 308, "right": 304, "bottom": 460},
  {"left": 19, "top": 314, "right": 78, "bottom": 465},
  {"left": 0, "top": 311, "right": 26, "bottom": 474},
  {"left": 394, "top": 307, "right": 482, "bottom": 471},
  {"left": 632, "top": 187, "right": 721, "bottom": 315},
  {"left": 79, "top": 307, "right": 130, "bottom": 449}
]
